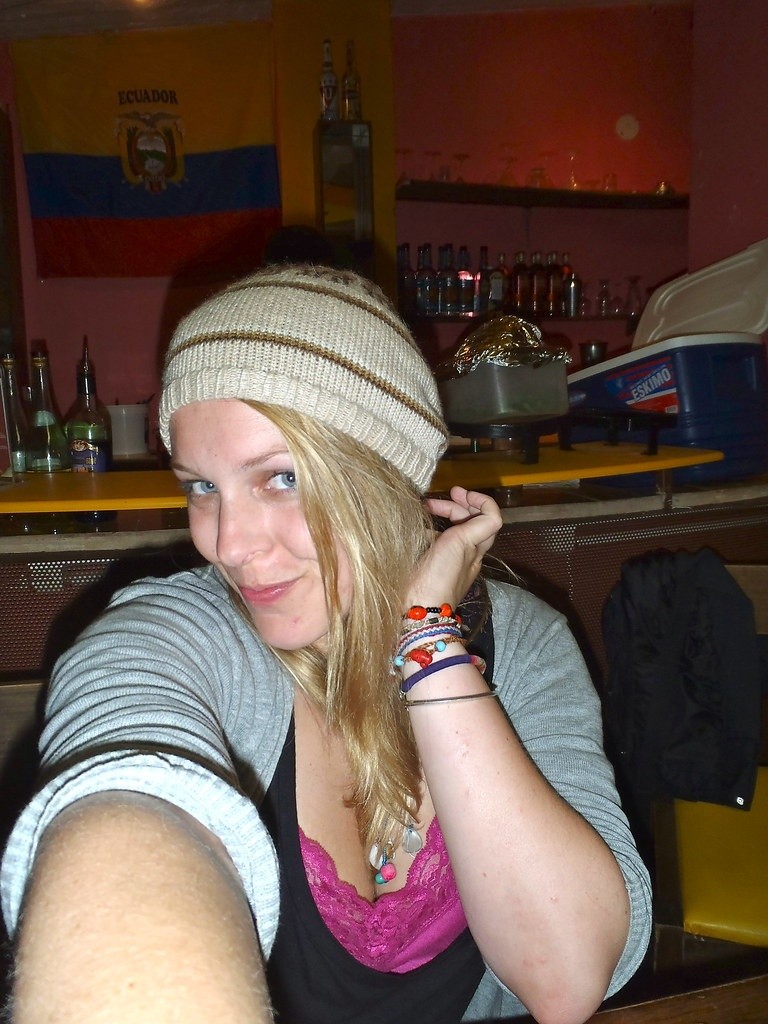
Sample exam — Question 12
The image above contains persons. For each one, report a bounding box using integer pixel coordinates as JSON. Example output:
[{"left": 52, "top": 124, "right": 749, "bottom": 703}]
[{"left": 2, "top": 262, "right": 654, "bottom": 1024}]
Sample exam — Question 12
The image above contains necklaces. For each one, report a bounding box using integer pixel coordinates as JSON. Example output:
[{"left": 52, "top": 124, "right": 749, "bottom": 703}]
[{"left": 369, "top": 819, "right": 422, "bottom": 884}]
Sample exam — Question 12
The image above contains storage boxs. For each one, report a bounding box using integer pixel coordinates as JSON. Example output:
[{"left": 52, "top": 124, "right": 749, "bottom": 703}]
[{"left": 560, "top": 236, "right": 767, "bottom": 491}]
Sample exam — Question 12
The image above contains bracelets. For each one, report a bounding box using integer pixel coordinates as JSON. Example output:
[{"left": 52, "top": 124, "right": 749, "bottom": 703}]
[{"left": 394, "top": 603, "right": 500, "bottom": 706}]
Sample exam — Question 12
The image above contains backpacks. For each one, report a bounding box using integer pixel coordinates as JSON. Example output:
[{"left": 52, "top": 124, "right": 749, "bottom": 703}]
[{"left": 598, "top": 545, "right": 765, "bottom": 811}]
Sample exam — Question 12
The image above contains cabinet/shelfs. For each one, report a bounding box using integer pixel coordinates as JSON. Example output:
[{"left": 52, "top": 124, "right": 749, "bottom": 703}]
[{"left": 392, "top": 179, "right": 689, "bottom": 326}]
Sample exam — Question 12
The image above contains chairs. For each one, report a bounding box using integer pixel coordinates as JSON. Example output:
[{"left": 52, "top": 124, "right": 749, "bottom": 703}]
[{"left": 650, "top": 559, "right": 768, "bottom": 996}]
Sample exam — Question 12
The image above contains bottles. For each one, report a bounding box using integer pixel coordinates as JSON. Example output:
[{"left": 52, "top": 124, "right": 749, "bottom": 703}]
[
  {"left": 64, "top": 348, "right": 108, "bottom": 473},
  {"left": 399, "top": 243, "right": 582, "bottom": 317},
  {"left": 314, "top": 41, "right": 375, "bottom": 240},
  {"left": 25, "top": 349, "right": 66, "bottom": 471},
  {"left": 3, "top": 354, "right": 25, "bottom": 472}
]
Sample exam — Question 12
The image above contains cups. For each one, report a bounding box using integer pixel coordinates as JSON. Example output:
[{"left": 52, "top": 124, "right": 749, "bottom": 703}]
[
  {"left": 583, "top": 276, "right": 643, "bottom": 315},
  {"left": 579, "top": 340, "right": 608, "bottom": 365},
  {"left": 398, "top": 149, "right": 616, "bottom": 191}
]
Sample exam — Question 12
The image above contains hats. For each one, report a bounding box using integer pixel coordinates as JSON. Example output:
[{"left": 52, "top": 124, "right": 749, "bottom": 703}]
[{"left": 158, "top": 263, "right": 450, "bottom": 495}]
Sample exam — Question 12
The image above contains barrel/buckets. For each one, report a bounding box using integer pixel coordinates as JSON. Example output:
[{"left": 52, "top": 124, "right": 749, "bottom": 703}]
[{"left": 106, "top": 404, "right": 148, "bottom": 456}]
